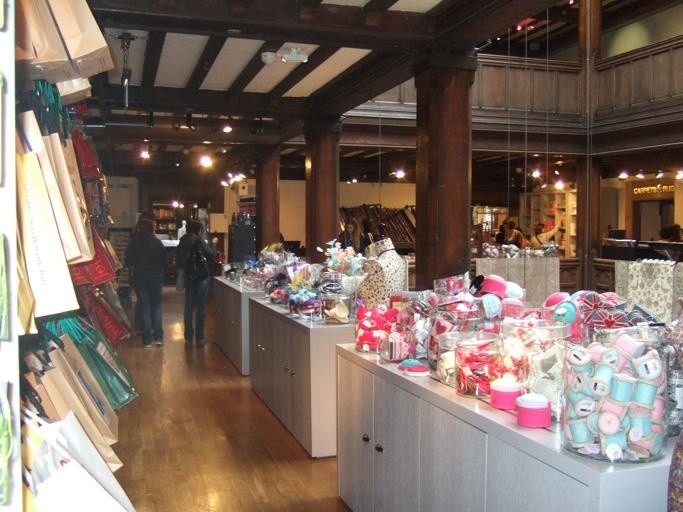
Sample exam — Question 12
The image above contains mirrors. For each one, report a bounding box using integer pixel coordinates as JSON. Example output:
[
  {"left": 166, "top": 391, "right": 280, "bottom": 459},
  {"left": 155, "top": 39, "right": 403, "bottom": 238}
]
[{"left": 597, "top": 163, "right": 683, "bottom": 261}]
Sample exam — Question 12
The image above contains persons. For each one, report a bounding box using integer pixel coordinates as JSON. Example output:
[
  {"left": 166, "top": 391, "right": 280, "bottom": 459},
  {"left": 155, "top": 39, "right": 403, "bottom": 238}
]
[
  {"left": 125, "top": 211, "right": 170, "bottom": 348},
  {"left": 530, "top": 216, "right": 563, "bottom": 247},
  {"left": 339, "top": 217, "right": 369, "bottom": 256},
  {"left": 496, "top": 220, "right": 529, "bottom": 249},
  {"left": 175, "top": 221, "right": 218, "bottom": 345}
]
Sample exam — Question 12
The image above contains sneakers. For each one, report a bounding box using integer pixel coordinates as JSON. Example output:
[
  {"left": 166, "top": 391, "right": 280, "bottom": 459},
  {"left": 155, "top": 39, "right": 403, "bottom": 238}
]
[
  {"left": 155, "top": 339, "right": 162, "bottom": 344},
  {"left": 144, "top": 343, "right": 152, "bottom": 348}
]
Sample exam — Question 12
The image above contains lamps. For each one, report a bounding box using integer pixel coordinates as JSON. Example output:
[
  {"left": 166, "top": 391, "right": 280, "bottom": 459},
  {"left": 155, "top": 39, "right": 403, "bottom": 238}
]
[
  {"left": 617, "top": 165, "right": 683, "bottom": 183},
  {"left": 135, "top": 111, "right": 265, "bottom": 169}
]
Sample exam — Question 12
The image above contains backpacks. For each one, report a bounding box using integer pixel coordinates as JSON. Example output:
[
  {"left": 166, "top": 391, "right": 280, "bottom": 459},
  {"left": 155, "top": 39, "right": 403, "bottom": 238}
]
[{"left": 186, "top": 240, "right": 208, "bottom": 281}]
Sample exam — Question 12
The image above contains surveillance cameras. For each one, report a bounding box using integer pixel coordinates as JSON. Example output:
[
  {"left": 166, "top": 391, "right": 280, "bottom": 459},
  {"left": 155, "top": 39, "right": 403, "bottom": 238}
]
[{"left": 261, "top": 51, "right": 309, "bottom": 65}]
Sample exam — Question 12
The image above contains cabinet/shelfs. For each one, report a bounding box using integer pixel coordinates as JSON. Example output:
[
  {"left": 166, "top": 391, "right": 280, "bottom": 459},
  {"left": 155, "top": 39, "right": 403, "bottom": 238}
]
[
  {"left": 519, "top": 187, "right": 577, "bottom": 259},
  {"left": 248, "top": 296, "right": 355, "bottom": 458},
  {"left": 336, "top": 357, "right": 420, "bottom": 512},
  {"left": 420, "top": 385, "right": 677, "bottom": 512},
  {"left": 212, "top": 276, "right": 264, "bottom": 376},
  {"left": 152, "top": 202, "right": 198, "bottom": 239}
]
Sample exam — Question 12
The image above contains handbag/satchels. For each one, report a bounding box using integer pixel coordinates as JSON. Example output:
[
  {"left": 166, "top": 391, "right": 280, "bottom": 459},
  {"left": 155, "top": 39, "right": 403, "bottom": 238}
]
[
  {"left": 24, "top": 278, "right": 139, "bottom": 512},
  {"left": 16, "top": 0, "right": 123, "bottom": 313}
]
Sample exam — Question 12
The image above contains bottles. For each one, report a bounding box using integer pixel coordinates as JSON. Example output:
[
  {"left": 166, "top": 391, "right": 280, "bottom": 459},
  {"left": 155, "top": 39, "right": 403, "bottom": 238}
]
[{"left": 297, "top": 271, "right": 683, "bottom": 464}]
